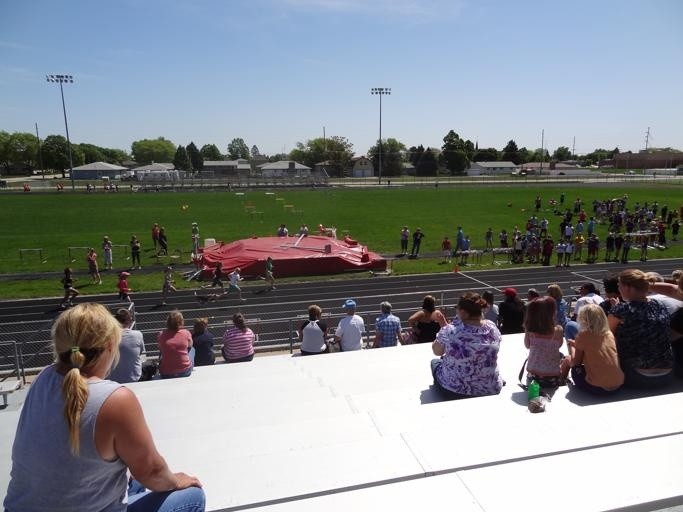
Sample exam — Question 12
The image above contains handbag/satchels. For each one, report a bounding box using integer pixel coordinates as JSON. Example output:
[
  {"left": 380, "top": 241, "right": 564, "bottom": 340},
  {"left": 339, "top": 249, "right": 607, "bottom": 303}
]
[
  {"left": 324, "top": 337, "right": 340, "bottom": 353},
  {"left": 138, "top": 360, "right": 161, "bottom": 381}
]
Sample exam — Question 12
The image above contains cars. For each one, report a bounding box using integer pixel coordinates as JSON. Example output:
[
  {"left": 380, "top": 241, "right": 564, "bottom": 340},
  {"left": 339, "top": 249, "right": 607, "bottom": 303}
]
[
  {"left": 525, "top": 168, "right": 535, "bottom": 176},
  {"left": 623, "top": 171, "right": 634, "bottom": 175}
]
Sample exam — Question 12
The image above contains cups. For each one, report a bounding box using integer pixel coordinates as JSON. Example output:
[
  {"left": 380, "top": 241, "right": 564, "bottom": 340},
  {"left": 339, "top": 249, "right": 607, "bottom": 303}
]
[{"left": 527, "top": 379, "right": 541, "bottom": 400}]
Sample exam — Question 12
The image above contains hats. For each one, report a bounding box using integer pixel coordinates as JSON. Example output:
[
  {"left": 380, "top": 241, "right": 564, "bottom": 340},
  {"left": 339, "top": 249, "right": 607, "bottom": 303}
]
[
  {"left": 500, "top": 287, "right": 516, "bottom": 295},
  {"left": 341, "top": 300, "right": 356, "bottom": 309}
]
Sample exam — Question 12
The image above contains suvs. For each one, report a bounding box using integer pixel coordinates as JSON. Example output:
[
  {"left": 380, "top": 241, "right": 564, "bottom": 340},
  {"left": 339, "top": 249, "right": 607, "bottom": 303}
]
[{"left": 512, "top": 169, "right": 525, "bottom": 176}]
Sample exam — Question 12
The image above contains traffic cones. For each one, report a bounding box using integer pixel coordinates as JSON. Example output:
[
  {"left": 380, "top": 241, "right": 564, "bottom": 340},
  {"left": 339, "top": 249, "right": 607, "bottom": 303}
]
[{"left": 453, "top": 264, "right": 459, "bottom": 273}]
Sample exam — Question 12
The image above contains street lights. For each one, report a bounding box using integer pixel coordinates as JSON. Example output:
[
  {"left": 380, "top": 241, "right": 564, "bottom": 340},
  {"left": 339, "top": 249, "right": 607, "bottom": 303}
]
[
  {"left": 45, "top": 72, "right": 77, "bottom": 190},
  {"left": 369, "top": 86, "right": 392, "bottom": 184}
]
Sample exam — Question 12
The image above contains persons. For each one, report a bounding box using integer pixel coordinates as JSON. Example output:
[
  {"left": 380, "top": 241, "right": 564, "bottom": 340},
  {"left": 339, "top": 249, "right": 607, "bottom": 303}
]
[
  {"left": 512, "top": 195, "right": 596, "bottom": 267},
  {"left": 565, "top": 271, "right": 682, "bottom": 341},
  {"left": 24, "top": 183, "right": 30, "bottom": 193},
  {"left": 86, "top": 183, "right": 119, "bottom": 193},
  {"left": 301, "top": 224, "right": 308, "bottom": 231},
  {"left": 86, "top": 223, "right": 255, "bottom": 383},
  {"left": 373, "top": 301, "right": 405, "bottom": 348},
  {"left": 442, "top": 226, "right": 508, "bottom": 265},
  {"left": 156, "top": 184, "right": 160, "bottom": 192},
  {"left": 256, "top": 256, "right": 276, "bottom": 290},
  {"left": 400, "top": 226, "right": 424, "bottom": 256},
  {"left": 57, "top": 183, "right": 64, "bottom": 192},
  {"left": 277, "top": 224, "right": 289, "bottom": 237},
  {"left": 567, "top": 303, "right": 624, "bottom": 397},
  {"left": 592, "top": 194, "right": 682, "bottom": 263},
  {"left": 130, "top": 183, "right": 133, "bottom": 192},
  {"left": 524, "top": 296, "right": 571, "bottom": 388},
  {"left": 608, "top": 269, "right": 675, "bottom": 389},
  {"left": 3, "top": 301, "right": 206, "bottom": 512},
  {"left": 300, "top": 305, "right": 330, "bottom": 356},
  {"left": 142, "top": 184, "right": 145, "bottom": 192},
  {"left": 482, "top": 285, "right": 568, "bottom": 334},
  {"left": 330, "top": 300, "right": 364, "bottom": 351},
  {"left": 60, "top": 268, "right": 80, "bottom": 307},
  {"left": 430, "top": 292, "right": 506, "bottom": 398},
  {"left": 404, "top": 295, "right": 448, "bottom": 344}
]
